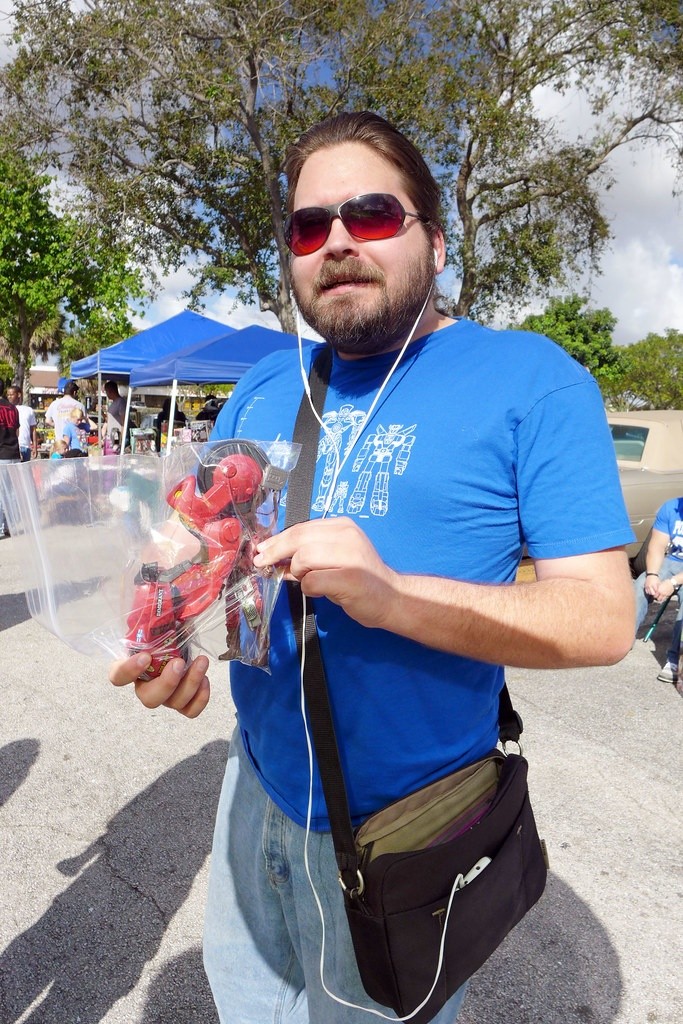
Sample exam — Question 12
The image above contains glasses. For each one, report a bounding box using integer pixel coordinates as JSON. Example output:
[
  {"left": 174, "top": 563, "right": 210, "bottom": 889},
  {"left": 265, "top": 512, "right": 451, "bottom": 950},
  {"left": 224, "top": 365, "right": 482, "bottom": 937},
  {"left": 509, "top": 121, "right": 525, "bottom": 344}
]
[{"left": 285, "top": 192, "right": 430, "bottom": 256}]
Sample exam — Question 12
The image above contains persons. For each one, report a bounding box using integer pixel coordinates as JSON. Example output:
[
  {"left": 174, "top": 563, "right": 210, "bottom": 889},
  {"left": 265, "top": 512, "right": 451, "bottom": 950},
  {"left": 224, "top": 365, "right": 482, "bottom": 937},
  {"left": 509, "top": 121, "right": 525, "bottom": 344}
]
[
  {"left": 634, "top": 496, "right": 683, "bottom": 683},
  {"left": 165, "top": 439, "right": 292, "bottom": 669},
  {"left": 110, "top": 114, "right": 636, "bottom": 1024},
  {"left": 0, "top": 377, "right": 220, "bottom": 540}
]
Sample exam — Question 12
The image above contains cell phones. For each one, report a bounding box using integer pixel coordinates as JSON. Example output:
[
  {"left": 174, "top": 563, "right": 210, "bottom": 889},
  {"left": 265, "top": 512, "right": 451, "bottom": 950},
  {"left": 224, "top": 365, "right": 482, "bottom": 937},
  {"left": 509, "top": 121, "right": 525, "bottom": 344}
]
[{"left": 460, "top": 856, "right": 491, "bottom": 889}]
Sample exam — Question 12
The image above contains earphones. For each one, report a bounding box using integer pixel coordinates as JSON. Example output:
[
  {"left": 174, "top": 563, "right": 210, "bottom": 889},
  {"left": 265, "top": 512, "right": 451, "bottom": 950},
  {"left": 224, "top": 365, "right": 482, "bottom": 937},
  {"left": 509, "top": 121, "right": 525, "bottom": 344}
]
[{"left": 433, "top": 248, "right": 439, "bottom": 266}]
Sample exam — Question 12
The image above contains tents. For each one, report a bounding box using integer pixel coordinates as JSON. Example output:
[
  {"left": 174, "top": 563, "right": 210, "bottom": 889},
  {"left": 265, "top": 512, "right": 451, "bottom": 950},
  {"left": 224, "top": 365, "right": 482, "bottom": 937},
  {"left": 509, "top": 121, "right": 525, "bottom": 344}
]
[{"left": 69, "top": 308, "right": 319, "bottom": 487}]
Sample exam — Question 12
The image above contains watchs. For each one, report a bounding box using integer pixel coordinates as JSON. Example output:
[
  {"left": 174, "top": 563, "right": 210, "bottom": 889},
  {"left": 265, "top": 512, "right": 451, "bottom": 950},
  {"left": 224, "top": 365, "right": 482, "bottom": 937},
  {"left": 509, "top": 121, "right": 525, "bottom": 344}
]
[{"left": 670, "top": 577, "right": 679, "bottom": 591}]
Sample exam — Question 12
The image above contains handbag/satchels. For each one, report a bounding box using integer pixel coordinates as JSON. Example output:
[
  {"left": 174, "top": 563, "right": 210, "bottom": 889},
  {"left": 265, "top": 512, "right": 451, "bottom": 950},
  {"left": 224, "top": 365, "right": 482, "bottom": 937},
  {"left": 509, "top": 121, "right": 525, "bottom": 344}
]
[
  {"left": 340, "top": 748, "right": 549, "bottom": 1024},
  {"left": 658, "top": 662, "right": 677, "bottom": 683}
]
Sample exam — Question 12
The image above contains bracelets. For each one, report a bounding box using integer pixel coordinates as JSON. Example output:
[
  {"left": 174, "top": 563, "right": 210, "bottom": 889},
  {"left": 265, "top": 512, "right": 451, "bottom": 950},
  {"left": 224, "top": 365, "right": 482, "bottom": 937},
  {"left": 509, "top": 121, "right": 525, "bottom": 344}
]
[{"left": 646, "top": 573, "right": 658, "bottom": 577}]
